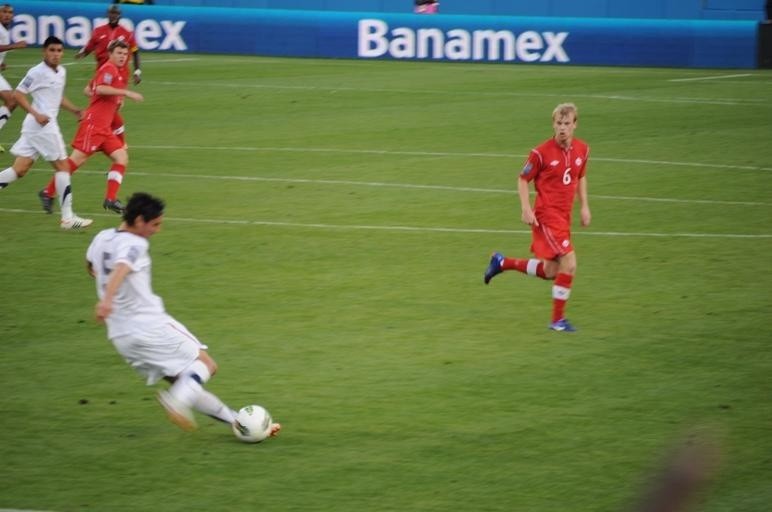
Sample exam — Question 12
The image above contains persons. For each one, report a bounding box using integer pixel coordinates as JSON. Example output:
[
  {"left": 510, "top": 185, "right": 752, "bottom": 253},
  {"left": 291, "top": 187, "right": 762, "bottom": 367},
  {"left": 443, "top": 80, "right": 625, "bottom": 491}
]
[
  {"left": 484, "top": 102, "right": 591, "bottom": 333},
  {"left": 85, "top": 193, "right": 281, "bottom": 441},
  {"left": 415, "top": 0, "right": 441, "bottom": 14},
  {"left": 0, "top": 0, "right": 153, "bottom": 230}
]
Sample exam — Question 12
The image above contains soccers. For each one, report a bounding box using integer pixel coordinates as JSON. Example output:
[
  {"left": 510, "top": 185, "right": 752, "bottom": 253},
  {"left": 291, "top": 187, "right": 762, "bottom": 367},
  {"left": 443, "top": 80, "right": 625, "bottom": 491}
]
[{"left": 232, "top": 405, "right": 274, "bottom": 443}]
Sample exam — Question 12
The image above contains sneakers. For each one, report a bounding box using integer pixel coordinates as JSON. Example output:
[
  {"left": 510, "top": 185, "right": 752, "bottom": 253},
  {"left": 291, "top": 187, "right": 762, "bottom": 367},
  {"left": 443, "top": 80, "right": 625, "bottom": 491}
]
[
  {"left": 483, "top": 253, "right": 503, "bottom": 284},
  {"left": 39, "top": 188, "right": 54, "bottom": 214},
  {"left": 158, "top": 388, "right": 196, "bottom": 430},
  {"left": 271, "top": 423, "right": 280, "bottom": 435},
  {"left": 104, "top": 199, "right": 126, "bottom": 212},
  {"left": 61, "top": 216, "right": 93, "bottom": 228},
  {"left": 550, "top": 319, "right": 575, "bottom": 331}
]
